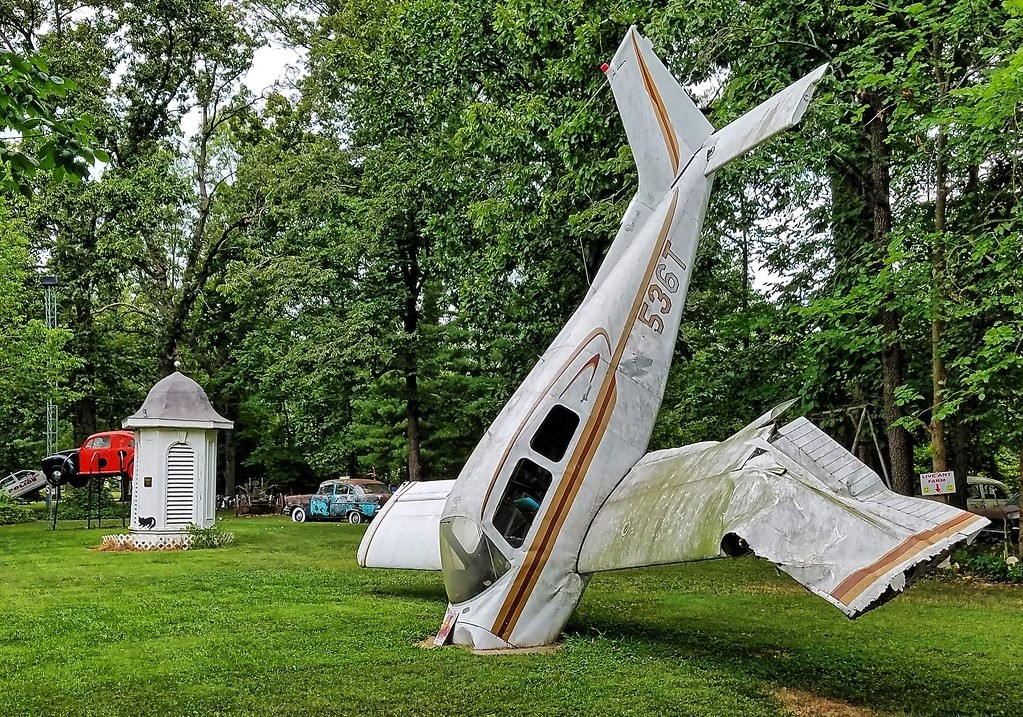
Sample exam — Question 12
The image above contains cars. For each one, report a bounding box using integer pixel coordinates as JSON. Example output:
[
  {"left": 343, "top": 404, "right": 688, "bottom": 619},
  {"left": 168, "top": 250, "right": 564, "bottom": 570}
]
[
  {"left": 41, "top": 430, "right": 135, "bottom": 487},
  {"left": 913, "top": 474, "right": 1020, "bottom": 523}
]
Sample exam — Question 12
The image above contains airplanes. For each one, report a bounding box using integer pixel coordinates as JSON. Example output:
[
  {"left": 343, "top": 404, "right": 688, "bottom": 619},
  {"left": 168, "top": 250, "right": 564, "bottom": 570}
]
[{"left": 356, "top": 21, "right": 994, "bottom": 648}]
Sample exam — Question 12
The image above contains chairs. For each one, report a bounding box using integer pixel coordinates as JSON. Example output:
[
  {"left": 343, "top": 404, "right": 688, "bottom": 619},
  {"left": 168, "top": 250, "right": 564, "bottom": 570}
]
[{"left": 513, "top": 497, "right": 538, "bottom": 522}]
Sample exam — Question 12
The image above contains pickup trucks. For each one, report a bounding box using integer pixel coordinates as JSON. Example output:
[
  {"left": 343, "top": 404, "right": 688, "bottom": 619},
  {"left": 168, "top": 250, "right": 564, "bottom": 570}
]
[{"left": 285, "top": 477, "right": 391, "bottom": 523}]
